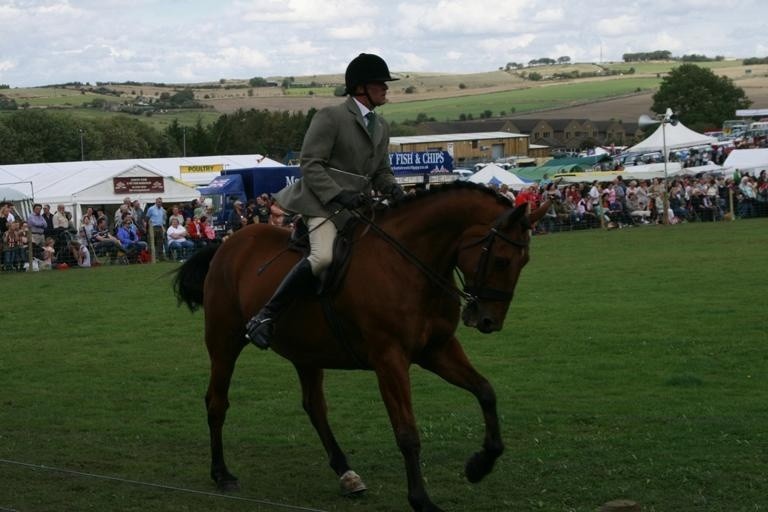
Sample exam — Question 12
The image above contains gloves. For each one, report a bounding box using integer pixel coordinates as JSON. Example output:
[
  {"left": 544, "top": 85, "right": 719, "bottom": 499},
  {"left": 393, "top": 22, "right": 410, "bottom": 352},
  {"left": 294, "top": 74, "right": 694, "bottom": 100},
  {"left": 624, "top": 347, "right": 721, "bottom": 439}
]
[{"left": 337, "top": 189, "right": 364, "bottom": 212}]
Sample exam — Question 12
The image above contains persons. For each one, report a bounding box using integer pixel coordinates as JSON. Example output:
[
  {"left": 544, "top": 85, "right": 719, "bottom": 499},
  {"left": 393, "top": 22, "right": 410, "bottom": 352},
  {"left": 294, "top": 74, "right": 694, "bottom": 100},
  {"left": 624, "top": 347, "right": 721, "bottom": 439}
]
[{"left": 244, "top": 51, "right": 403, "bottom": 348}]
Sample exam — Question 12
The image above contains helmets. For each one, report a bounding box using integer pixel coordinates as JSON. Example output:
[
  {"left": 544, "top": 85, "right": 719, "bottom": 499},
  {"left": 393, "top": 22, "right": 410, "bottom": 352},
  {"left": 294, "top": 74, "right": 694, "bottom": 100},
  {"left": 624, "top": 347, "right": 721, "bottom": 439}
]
[{"left": 344, "top": 51, "right": 400, "bottom": 85}]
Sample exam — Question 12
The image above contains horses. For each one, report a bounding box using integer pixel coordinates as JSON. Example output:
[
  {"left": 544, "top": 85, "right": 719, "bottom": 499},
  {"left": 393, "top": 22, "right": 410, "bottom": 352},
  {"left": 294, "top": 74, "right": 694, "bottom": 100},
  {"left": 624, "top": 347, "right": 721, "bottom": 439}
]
[{"left": 155, "top": 179, "right": 554, "bottom": 512}]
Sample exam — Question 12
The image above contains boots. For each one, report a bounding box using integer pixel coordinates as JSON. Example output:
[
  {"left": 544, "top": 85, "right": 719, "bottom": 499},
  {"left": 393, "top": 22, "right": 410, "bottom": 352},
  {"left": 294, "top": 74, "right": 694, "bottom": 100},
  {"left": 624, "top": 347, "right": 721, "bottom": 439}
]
[{"left": 244, "top": 259, "right": 316, "bottom": 352}]
